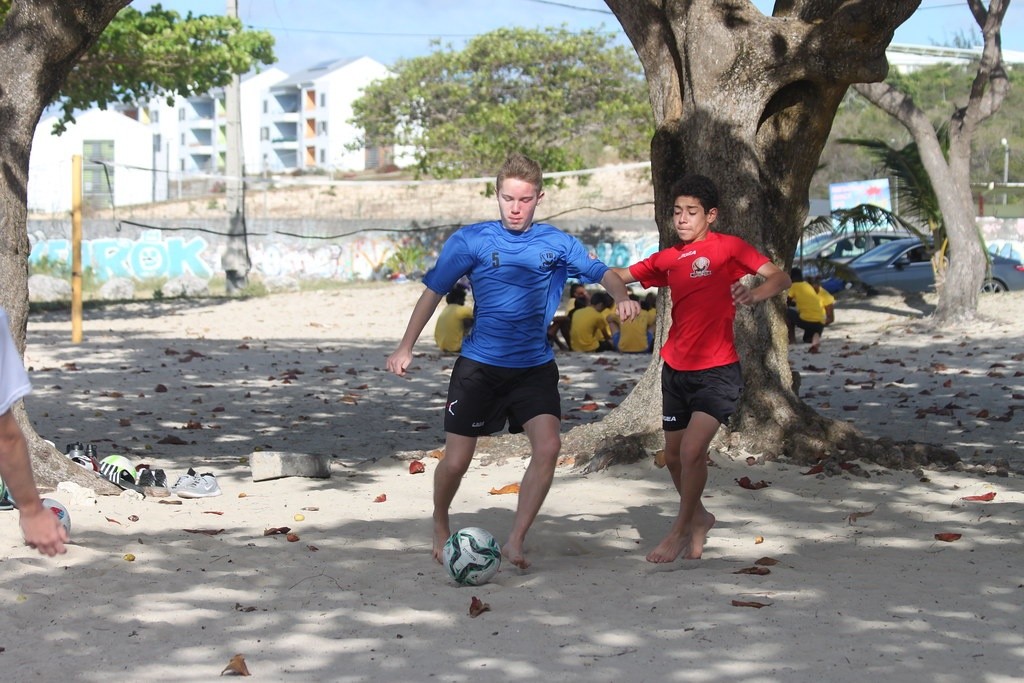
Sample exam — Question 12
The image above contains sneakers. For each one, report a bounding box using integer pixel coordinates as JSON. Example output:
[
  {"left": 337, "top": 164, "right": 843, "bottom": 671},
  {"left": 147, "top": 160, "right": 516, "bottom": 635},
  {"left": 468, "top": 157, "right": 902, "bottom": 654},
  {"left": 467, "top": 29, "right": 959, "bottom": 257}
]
[
  {"left": 137, "top": 470, "right": 154, "bottom": 497},
  {"left": 177, "top": 472, "right": 222, "bottom": 497},
  {"left": 169, "top": 472, "right": 197, "bottom": 494},
  {"left": 153, "top": 469, "right": 170, "bottom": 496}
]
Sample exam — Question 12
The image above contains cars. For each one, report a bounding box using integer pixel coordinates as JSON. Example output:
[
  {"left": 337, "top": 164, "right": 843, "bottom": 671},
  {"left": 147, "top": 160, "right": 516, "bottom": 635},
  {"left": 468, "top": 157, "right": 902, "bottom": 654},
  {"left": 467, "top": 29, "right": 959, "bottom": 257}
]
[
  {"left": 800, "top": 237, "right": 1023, "bottom": 298},
  {"left": 792, "top": 229, "right": 921, "bottom": 270}
]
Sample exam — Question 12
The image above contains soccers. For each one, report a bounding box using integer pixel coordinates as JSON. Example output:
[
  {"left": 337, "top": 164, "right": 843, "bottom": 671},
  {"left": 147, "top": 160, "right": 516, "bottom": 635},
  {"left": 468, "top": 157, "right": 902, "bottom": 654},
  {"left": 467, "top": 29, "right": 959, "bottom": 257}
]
[
  {"left": 443, "top": 525, "right": 501, "bottom": 587},
  {"left": 99, "top": 454, "right": 136, "bottom": 483},
  {"left": 18, "top": 497, "right": 71, "bottom": 544}
]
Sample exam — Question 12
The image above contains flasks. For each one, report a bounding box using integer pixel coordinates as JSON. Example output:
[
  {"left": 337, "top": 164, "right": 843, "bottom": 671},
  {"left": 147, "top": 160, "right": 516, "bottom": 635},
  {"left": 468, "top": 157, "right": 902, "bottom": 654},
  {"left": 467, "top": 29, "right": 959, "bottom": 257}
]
[
  {"left": 85, "top": 444, "right": 97, "bottom": 460},
  {"left": 66, "top": 441, "right": 84, "bottom": 458}
]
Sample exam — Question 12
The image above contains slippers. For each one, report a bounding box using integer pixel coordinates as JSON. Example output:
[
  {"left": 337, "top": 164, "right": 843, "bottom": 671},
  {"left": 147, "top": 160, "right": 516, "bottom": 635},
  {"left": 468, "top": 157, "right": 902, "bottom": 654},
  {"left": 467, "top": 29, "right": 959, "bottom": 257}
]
[
  {"left": 92, "top": 457, "right": 118, "bottom": 478},
  {"left": 104, "top": 469, "right": 145, "bottom": 496}
]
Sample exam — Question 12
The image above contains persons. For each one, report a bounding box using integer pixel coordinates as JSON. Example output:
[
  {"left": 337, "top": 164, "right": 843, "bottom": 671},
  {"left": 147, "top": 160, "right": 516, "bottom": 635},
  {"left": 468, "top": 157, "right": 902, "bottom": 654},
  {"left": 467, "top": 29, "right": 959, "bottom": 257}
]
[
  {"left": 569, "top": 292, "right": 613, "bottom": 353},
  {"left": 434, "top": 290, "right": 473, "bottom": 354},
  {"left": 0, "top": 305, "right": 71, "bottom": 556},
  {"left": 385, "top": 154, "right": 641, "bottom": 571},
  {"left": 806, "top": 274, "right": 836, "bottom": 327},
  {"left": 785, "top": 267, "right": 826, "bottom": 354},
  {"left": 568, "top": 296, "right": 588, "bottom": 319},
  {"left": 594, "top": 286, "right": 656, "bottom": 343},
  {"left": 606, "top": 295, "right": 656, "bottom": 354},
  {"left": 566, "top": 283, "right": 591, "bottom": 314},
  {"left": 608, "top": 173, "right": 792, "bottom": 564}
]
